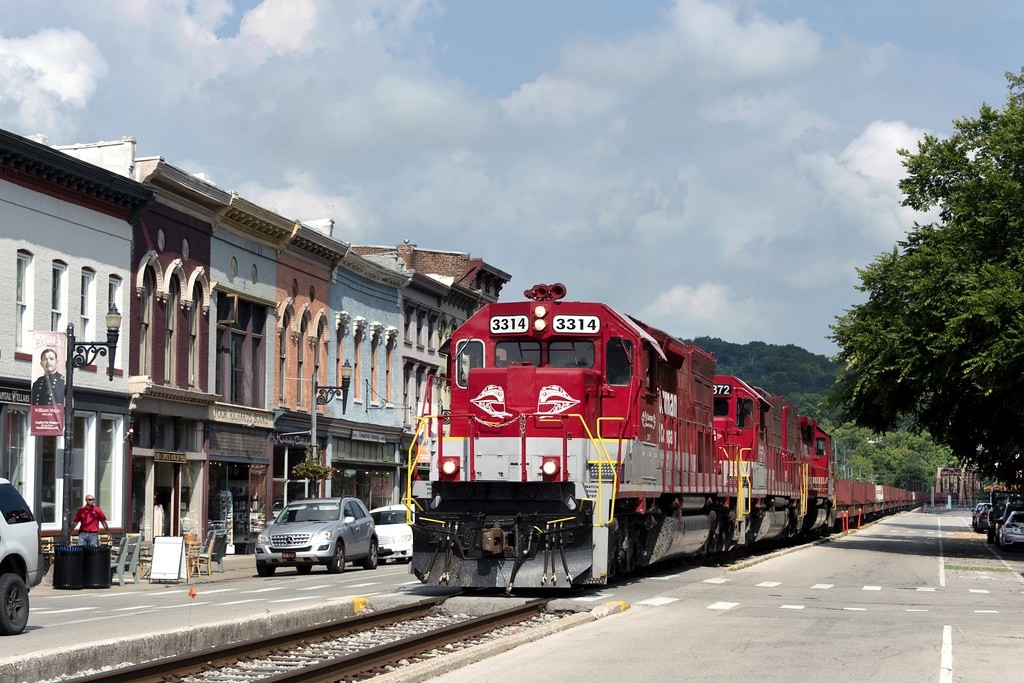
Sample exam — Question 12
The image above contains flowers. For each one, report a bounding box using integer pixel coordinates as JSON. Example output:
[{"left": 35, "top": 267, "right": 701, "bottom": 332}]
[{"left": 291, "top": 462, "right": 335, "bottom": 480}]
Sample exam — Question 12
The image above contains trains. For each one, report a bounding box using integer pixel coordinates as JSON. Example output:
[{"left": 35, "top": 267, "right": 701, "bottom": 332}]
[{"left": 401, "top": 283, "right": 957, "bottom": 590}]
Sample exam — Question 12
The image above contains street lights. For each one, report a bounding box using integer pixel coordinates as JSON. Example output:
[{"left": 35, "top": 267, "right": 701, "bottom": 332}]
[
  {"left": 62, "top": 302, "right": 122, "bottom": 547},
  {"left": 310, "top": 358, "right": 353, "bottom": 497}
]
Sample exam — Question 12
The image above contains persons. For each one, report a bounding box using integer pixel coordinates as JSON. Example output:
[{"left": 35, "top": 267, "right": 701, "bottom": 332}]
[
  {"left": 987, "top": 497, "right": 1003, "bottom": 543},
  {"left": 31, "top": 349, "right": 67, "bottom": 405},
  {"left": 70, "top": 494, "right": 112, "bottom": 546}
]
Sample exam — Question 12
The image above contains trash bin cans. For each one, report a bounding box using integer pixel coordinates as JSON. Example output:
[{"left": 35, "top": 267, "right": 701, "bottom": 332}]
[
  {"left": 53, "top": 544, "right": 83, "bottom": 589},
  {"left": 80, "top": 545, "right": 113, "bottom": 588}
]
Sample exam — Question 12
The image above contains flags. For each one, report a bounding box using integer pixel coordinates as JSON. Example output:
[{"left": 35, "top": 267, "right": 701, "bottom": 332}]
[{"left": 417, "top": 416, "right": 431, "bottom": 463}]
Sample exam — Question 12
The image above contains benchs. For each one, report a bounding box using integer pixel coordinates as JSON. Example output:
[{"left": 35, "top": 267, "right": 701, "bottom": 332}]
[{"left": 111, "top": 535, "right": 142, "bottom": 588}]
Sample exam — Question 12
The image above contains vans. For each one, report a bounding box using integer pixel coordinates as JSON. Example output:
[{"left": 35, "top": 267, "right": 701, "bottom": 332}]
[{"left": 369, "top": 503, "right": 417, "bottom": 565}]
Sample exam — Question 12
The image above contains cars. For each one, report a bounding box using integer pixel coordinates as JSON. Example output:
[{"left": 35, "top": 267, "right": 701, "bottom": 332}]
[
  {"left": 970, "top": 503, "right": 992, "bottom": 533},
  {"left": 986, "top": 490, "right": 1024, "bottom": 553}
]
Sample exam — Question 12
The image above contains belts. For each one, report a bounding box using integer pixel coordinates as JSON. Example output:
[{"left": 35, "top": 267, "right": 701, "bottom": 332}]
[{"left": 80, "top": 531, "right": 97, "bottom": 533}]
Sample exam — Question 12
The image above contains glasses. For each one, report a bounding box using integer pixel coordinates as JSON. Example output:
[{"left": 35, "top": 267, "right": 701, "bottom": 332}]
[{"left": 87, "top": 499, "right": 94, "bottom": 501}]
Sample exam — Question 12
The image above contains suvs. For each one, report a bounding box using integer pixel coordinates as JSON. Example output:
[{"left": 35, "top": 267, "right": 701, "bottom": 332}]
[
  {"left": 254, "top": 497, "right": 379, "bottom": 577},
  {"left": 0, "top": 477, "right": 45, "bottom": 636}
]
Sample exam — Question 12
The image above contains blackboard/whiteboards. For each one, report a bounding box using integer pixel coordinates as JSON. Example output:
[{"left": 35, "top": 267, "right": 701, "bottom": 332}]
[{"left": 150, "top": 535, "right": 189, "bottom": 580}]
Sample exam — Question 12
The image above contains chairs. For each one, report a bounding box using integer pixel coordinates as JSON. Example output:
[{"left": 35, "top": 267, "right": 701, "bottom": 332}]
[{"left": 185, "top": 528, "right": 217, "bottom": 578}]
